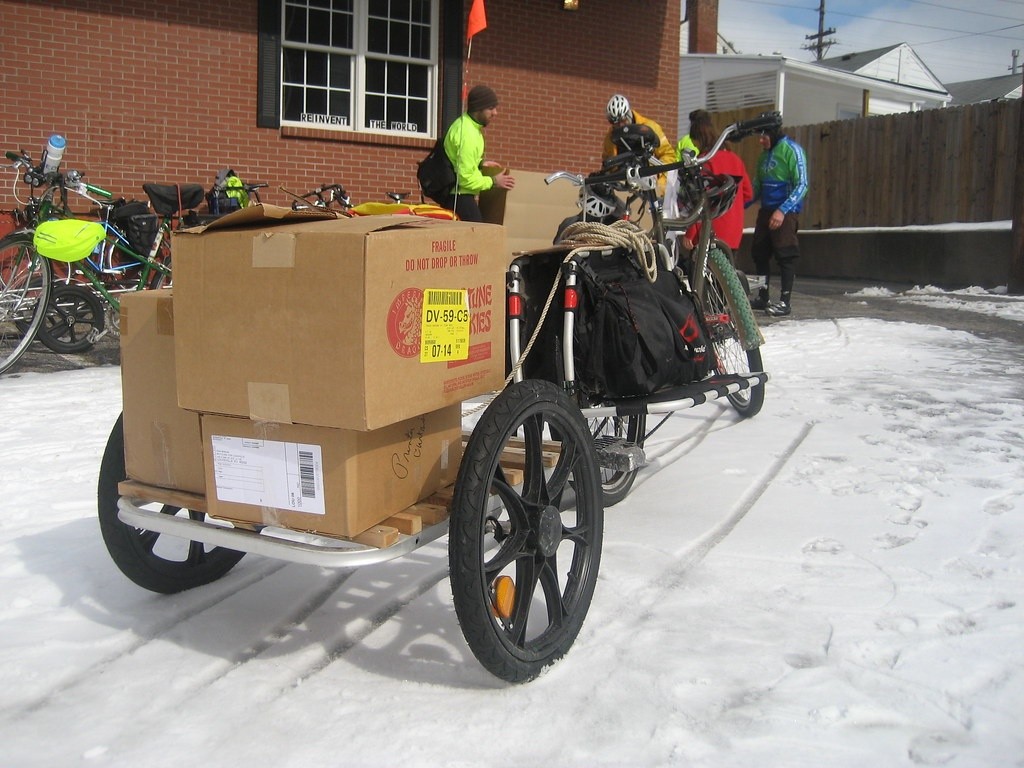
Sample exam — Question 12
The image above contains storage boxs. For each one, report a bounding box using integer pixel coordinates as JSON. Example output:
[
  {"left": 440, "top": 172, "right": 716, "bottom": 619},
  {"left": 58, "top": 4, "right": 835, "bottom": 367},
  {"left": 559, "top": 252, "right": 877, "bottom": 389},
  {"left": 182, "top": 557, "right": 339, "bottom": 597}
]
[
  {"left": 477, "top": 167, "right": 654, "bottom": 270},
  {"left": 170, "top": 202, "right": 506, "bottom": 432},
  {"left": 201, "top": 402, "right": 462, "bottom": 542},
  {"left": 119, "top": 288, "right": 205, "bottom": 495}
]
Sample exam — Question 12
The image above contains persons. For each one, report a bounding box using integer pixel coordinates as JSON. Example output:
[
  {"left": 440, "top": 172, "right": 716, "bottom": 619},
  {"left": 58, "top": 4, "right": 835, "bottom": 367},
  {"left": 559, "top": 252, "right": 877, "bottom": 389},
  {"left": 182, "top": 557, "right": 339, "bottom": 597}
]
[
  {"left": 439, "top": 86, "right": 514, "bottom": 222},
  {"left": 603, "top": 94, "right": 808, "bottom": 316}
]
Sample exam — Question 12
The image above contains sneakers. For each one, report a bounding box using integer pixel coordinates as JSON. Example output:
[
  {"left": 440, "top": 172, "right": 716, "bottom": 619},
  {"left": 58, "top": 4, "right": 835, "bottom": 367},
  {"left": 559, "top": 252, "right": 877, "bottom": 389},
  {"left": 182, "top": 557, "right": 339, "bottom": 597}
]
[
  {"left": 746, "top": 296, "right": 769, "bottom": 310},
  {"left": 767, "top": 301, "right": 788, "bottom": 317}
]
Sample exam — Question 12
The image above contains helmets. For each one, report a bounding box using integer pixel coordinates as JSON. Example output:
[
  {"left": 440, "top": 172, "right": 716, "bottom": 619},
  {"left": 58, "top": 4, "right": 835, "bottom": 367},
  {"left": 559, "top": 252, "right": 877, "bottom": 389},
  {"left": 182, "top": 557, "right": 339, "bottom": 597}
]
[
  {"left": 576, "top": 185, "right": 618, "bottom": 217},
  {"left": 676, "top": 175, "right": 743, "bottom": 222},
  {"left": 754, "top": 110, "right": 783, "bottom": 131},
  {"left": 605, "top": 94, "right": 633, "bottom": 122}
]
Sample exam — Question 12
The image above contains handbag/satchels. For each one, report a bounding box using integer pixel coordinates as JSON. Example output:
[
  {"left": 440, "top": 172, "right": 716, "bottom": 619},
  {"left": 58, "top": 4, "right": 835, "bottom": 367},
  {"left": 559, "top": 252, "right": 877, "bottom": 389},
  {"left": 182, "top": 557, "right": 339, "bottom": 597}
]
[
  {"left": 205, "top": 168, "right": 250, "bottom": 213},
  {"left": 589, "top": 270, "right": 714, "bottom": 394},
  {"left": 418, "top": 139, "right": 457, "bottom": 204},
  {"left": 32, "top": 218, "right": 106, "bottom": 262}
]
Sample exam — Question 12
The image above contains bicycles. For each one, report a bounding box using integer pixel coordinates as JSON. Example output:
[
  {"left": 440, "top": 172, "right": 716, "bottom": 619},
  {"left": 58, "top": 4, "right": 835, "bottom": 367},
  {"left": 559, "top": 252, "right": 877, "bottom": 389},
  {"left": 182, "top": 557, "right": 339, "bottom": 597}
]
[{"left": 0, "top": 146, "right": 430, "bottom": 376}]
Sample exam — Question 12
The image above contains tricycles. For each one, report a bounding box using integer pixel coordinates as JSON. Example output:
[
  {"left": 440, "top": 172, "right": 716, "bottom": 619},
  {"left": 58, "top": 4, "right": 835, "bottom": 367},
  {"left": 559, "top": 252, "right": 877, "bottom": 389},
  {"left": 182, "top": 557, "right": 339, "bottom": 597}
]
[{"left": 96, "top": 109, "right": 783, "bottom": 685}]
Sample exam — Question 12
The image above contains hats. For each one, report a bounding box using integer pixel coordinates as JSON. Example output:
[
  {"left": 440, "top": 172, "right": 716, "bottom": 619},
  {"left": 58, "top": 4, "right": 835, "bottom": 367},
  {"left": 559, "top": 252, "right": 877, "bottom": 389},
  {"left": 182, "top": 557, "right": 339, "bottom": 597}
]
[{"left": 467, "top": 85, "right": 500, "bottom": 112}]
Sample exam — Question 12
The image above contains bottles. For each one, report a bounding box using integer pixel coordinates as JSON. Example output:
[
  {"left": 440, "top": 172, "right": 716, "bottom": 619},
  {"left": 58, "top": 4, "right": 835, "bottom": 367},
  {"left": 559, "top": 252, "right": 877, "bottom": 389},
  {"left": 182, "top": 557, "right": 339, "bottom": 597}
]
[{"left": 43, "top": 135, "right": 66, "bottom": 175}]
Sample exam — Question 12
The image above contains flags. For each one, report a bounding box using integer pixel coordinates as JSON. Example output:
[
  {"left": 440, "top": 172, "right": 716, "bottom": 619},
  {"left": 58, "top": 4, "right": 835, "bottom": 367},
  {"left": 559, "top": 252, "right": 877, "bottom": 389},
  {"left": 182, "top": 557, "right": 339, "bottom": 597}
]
[{"left": 465, "top": 0, "right": 487, "bottom": 44}]
